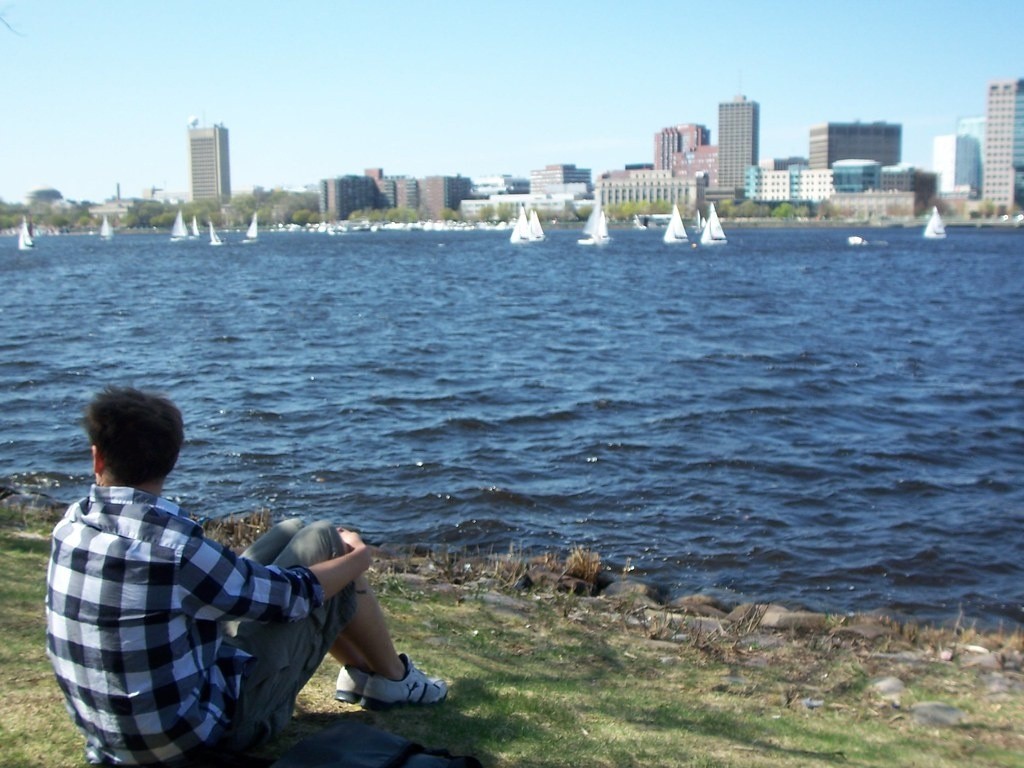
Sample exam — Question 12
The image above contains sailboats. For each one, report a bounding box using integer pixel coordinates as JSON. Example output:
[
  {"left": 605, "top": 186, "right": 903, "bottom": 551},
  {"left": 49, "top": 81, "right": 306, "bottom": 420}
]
[
  {"left": 170, "top": 209, "right": 203, "bottom": 242},
  {"left": 509, "top": 204, "right": 546, "bottom": 245},
  {"left": 700, "top": 202, "right": 729, "bottom": 247},
  {"left": 18, "top": 213, "right": 35, "bottom": 250},
  {"left": 99, "top": 212, "right": 114, "bottom": 239},
  {"left": 691, "top": 211, "right": 707, "bottom": 234},
  {"left": 241, "top": 211, "right": 260, "bottom": 244},
  {"left": 923, "top": 205, "right": 947, "bottom": 242},
  {"left": 208, "top": 221, "right": 227, "bottom": 247},
  {"left": 663, "top": 205, "right": 690, "bottom": 243},
  {"left": 576, "top": 195, "right": 614, "bottom": 245}
]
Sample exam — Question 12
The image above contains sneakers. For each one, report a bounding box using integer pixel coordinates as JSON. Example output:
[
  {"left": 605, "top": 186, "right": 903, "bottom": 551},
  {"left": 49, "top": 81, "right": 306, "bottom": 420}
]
[
  {"left": 362, "top": 654, "right": 447, "bottom": 712},
  {"left": 334, "top": 666, "right": 370, "bottom": 702}
]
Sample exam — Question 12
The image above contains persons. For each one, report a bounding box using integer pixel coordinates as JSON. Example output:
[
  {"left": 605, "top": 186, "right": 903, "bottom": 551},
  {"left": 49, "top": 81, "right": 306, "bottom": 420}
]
[{"left": 44, "top": 384, "right": 448, "bottom": 768}]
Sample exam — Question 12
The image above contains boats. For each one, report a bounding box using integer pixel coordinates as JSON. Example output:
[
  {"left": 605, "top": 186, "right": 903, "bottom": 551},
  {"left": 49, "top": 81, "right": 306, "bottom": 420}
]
[{"left": 848, "top": 236, "right": 868, "bottom": 246}]
